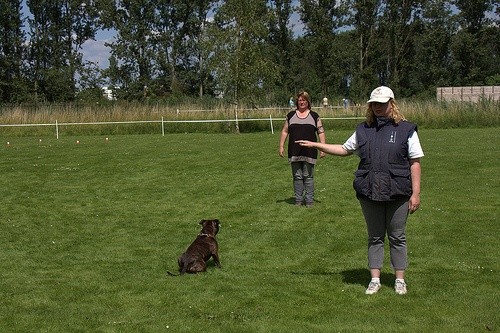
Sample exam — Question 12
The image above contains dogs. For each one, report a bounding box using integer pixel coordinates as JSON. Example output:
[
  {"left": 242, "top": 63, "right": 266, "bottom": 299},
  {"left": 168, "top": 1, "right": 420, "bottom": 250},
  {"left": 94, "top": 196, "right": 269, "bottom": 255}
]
[{"left": 166, "top": 219, "right": 222, "bottom": 277}]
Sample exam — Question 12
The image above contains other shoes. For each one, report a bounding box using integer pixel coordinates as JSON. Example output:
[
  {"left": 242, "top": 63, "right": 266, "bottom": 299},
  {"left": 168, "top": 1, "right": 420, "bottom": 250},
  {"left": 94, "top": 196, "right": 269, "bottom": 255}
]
[
  {"left": 306, "top": 201, "right": 313, "bottom": 208},
  {"left": 295, "top": 200, "right": 303, "bottom": 206}
]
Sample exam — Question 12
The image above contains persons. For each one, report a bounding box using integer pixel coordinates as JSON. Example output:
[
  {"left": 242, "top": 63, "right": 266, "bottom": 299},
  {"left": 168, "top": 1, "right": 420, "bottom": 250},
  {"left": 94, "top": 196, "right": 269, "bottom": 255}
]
[
  {"left": 294, "top": 86, "right": 424, "bottom": 295},
  {"left": 279, "top": 91, "right": 326, "bottom": 207}
]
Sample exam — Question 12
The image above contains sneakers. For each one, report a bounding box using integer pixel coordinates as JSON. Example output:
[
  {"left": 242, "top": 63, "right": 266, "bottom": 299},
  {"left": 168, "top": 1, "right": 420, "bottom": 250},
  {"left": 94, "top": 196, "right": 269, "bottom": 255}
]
[
  {"left": 365, "top": 281, "right": 381, "bottom": 294},
  {"left": 395, "top": 278, "right": 407, "bottom": 295}
]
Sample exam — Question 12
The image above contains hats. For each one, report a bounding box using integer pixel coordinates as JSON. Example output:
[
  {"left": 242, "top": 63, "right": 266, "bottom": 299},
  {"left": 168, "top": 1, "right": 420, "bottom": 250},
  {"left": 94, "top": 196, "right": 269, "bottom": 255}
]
[{"left": 367, "top": 85, "right": 395, "bottom": 103}]
